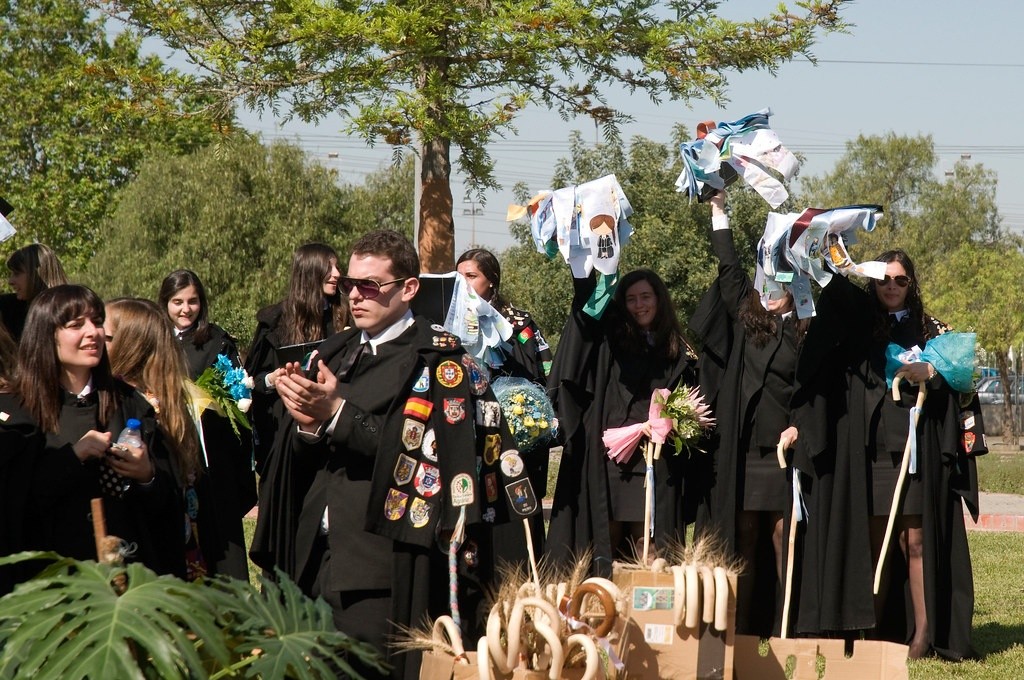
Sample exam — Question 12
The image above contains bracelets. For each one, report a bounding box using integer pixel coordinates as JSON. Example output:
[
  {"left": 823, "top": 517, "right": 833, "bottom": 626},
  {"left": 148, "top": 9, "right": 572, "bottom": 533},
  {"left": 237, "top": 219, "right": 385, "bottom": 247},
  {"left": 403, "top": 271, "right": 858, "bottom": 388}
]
[
  {"left": 139, "top": 463, "right": 156, "bottom": 486},
  {"left": 930, "top": 370, "right": 938, "bottom": 380}
]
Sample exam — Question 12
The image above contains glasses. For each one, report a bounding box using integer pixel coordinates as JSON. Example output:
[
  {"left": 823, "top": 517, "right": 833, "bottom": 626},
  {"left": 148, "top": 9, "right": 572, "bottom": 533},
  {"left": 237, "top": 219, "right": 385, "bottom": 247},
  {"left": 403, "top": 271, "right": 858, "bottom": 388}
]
[
  {"left": 337, "top": 275, "right": 412, "bottom": 299},
  {"left": 877, "top": 274, "right": 909, "bottom": 287}
]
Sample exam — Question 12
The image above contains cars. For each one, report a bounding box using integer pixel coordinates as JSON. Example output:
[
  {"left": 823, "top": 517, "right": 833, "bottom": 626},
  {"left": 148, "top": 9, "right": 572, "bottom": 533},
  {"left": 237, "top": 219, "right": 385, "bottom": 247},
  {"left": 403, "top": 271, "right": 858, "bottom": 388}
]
[{"left": 975, "top": 368, "right": 1024, "bottom": 405}]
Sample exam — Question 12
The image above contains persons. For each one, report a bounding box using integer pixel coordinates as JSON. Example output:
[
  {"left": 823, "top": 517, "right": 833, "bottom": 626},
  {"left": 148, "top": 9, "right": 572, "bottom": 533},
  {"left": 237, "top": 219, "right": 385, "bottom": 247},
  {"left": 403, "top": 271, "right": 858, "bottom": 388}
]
[{"left": 0, "top": 191, "right": 989, "bottom": 680}]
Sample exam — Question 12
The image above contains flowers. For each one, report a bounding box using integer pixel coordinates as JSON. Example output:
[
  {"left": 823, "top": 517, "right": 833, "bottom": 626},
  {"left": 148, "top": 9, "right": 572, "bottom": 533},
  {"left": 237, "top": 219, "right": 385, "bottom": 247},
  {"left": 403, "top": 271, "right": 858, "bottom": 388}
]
[
  {"left": 653, "top": 374, "right": 719, "bottom": 461},
  {"left": 193, "top": 352, "right": 255, "bottom": 444},
  {"left": 924, "top": 327, "right": 988, "bottom": 394},
  {"left": 501, "top": 390, "right": 558, "bottom": 451}
]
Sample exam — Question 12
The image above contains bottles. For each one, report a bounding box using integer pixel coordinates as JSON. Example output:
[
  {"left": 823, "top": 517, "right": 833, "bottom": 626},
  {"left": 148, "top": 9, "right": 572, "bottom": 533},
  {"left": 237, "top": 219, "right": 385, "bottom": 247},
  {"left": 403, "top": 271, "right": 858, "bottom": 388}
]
[{"left": 116, "top": 418, "right": 142, "bottom": 449}]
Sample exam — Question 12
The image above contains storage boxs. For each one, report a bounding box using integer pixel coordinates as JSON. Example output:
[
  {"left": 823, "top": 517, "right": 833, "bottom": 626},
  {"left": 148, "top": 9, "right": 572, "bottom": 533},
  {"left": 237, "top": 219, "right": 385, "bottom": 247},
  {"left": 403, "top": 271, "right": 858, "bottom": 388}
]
[{"left": 420, "top": 562, "right": 910, "bottom": 680}]
